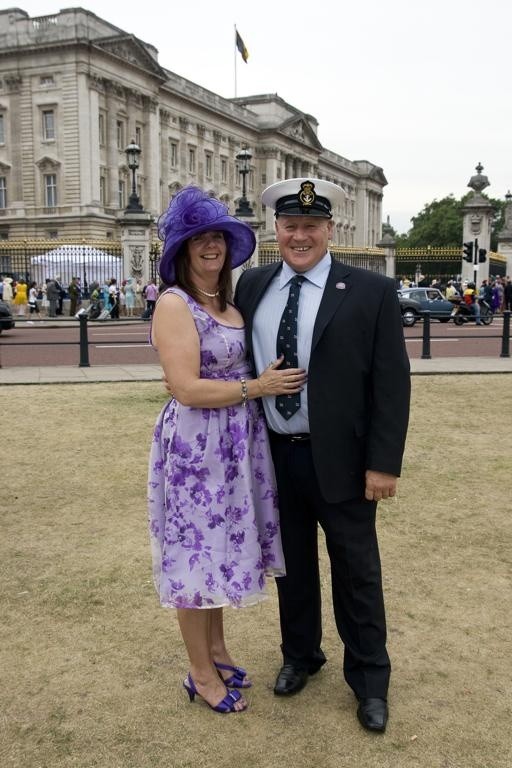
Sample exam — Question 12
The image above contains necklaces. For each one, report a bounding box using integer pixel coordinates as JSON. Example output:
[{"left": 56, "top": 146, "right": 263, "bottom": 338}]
[{"left": 191, "top": 282, "right": 225, "bottom": 303}]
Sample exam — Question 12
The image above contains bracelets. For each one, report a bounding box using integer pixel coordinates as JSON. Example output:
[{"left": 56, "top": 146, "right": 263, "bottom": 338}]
[{"left": 236, "top": 375, "right": 251, "bottom": 409}]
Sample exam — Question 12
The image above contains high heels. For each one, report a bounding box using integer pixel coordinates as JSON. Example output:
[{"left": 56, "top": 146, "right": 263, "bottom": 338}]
[
  {"left": 215, "top": 663, "right": 252, "bottom": 688},
  {"left": 183, "top": 672, "right": 247, "bottom": 713}
]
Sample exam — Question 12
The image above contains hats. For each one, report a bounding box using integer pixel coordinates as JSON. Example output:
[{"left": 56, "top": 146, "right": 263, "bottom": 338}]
[
  {"left": 260, "top": 177, "right": 347, "bottom": 218},
  {"left": 159, "top": 187, "right": 257, "bottom": 287}
]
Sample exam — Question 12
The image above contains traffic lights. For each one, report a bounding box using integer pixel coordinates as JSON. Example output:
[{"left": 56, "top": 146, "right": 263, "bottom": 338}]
[
  {"left": 462, "top": 241, "right": 472, "bottom": 264},
  {"left": 479, "top": 250, "right": 486, "bottom": 263}
]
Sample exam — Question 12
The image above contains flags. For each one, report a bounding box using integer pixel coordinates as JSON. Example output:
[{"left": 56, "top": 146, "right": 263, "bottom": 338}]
[{"left": 235, "top": 29, "right": 248, "bottom": 64}]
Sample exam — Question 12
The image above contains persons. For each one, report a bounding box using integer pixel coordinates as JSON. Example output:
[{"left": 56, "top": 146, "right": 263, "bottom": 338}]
[
  {"left": 228, "top": 173, "right": 414, "bottom": 737},
  {"left": 392, "top": 272, "right": 512, "bottom": 316},
  {"left": 141, "top": 181, "right": 313, "bottom": 720},
  {"left": 462, "top": 280, "right": 487, "bottom": 326},
  {"left": 0, "top": 270, "right": 165, "bottom": 323}
]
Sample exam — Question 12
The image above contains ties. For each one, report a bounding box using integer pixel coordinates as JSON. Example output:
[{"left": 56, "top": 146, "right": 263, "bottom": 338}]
[{"left": 274, "top": 277, "right": 306, "bottom": 420}]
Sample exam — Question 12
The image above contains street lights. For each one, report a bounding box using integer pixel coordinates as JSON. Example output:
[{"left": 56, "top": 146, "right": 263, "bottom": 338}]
[
  {"left": 150, "top": 244, "right": 159, "bottom": 315},
  {"left": 124, "top": 138, "right": 149, "bottom": 215},
  {"left": 233, "top": 141, "right": 255, "bottom": 217}
]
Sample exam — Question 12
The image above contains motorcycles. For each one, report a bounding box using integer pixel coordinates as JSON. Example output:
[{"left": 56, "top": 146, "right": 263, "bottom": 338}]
[{"left": 448, "top": 290, "right": 493, "bottom": 326}]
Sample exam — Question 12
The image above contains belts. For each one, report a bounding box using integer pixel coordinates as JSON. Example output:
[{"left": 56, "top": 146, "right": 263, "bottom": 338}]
[{"left": 287, "top": 434, "right": 310, "bottom": 443}]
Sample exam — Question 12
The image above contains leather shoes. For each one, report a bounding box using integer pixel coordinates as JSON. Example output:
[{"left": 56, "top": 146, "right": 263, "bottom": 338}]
[
  {"left": 356, "top": 696, "right": 389, "bottom": 732},
  {"left": 273, "top": 664, "right": 309, "bottom": 696}
]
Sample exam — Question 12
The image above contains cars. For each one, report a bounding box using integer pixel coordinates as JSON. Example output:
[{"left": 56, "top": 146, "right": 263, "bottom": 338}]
[
  {"left": 397, "top": 287, "right": 467, "bottom": 326},
  {"left": 0, "top": 298, "right": 15, "bottom": 336},
  {"left": 397, "top": 292, "right": 423, "bottom": 327}
]
